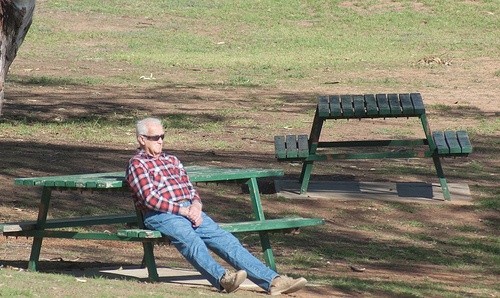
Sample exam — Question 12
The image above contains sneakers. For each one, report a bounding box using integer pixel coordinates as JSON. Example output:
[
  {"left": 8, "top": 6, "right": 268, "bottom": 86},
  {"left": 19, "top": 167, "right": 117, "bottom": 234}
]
[
  {"left": 220, "top": 269, "right": 247, "bottom": 293},
  {"left": 271, "top": 275, "right": 307, "bottom": 295}
]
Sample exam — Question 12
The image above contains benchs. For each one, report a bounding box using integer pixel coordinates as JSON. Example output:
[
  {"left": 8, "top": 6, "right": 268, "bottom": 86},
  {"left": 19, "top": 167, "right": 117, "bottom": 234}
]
[
  {"left": 434, "top": 130, "right": 472, "bottom": 155},
  {"left": 1, "top": 213, "right": 146, "bottom": 271},
  {"left": 274, "top": 134, "right": 309, "bottom": 161},
  {"left": 118, "top": 217, "right": 326, "bottom": 284}
]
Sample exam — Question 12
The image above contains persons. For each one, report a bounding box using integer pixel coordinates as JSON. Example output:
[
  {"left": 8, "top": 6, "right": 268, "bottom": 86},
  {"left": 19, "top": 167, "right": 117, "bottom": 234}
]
[{"left": 126, "top": 118, "right": 307, "bottom": 297}]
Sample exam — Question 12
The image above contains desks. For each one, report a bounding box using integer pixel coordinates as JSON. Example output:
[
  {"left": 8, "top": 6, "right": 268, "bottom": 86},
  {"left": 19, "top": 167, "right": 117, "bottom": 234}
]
[
  {"left": 299, "top": 93, "right": 453, "bottom": 200},
  {"left": 15, "top": 166, "right": 283, "bottom": 272}
]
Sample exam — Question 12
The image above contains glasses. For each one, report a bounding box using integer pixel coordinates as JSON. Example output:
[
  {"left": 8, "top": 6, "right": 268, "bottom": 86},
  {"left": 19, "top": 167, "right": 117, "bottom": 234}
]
[{"left": 143, "top": 134, "right": 164, "bottom": 141}]
